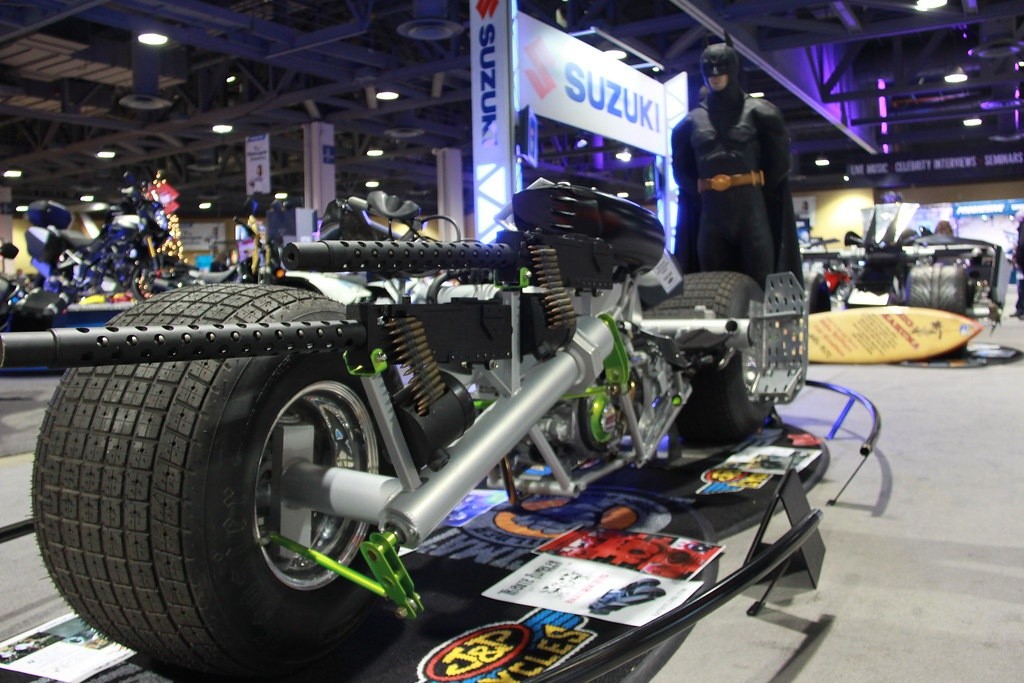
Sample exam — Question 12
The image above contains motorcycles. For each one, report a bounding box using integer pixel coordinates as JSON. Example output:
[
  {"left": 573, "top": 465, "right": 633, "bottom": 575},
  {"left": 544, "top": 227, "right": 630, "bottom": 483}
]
[
  {"left": 24, "top": 169, "right": 175, "bottom": 298},
  {"left": 0, "top": 204, "right": 1016, "bottom": 683}
]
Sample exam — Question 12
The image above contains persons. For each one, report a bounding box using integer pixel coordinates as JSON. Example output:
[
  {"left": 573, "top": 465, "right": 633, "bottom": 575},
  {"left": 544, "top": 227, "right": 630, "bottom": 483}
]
[
  {"left": 1010, "top": 210, "right": 1024, "bottom": 320},
  {"left": 935, "top": 220, "right": 954, "bottom": 239},
  {"left": 670, "top": 29, "right": 803, "bottom": 294}
]
